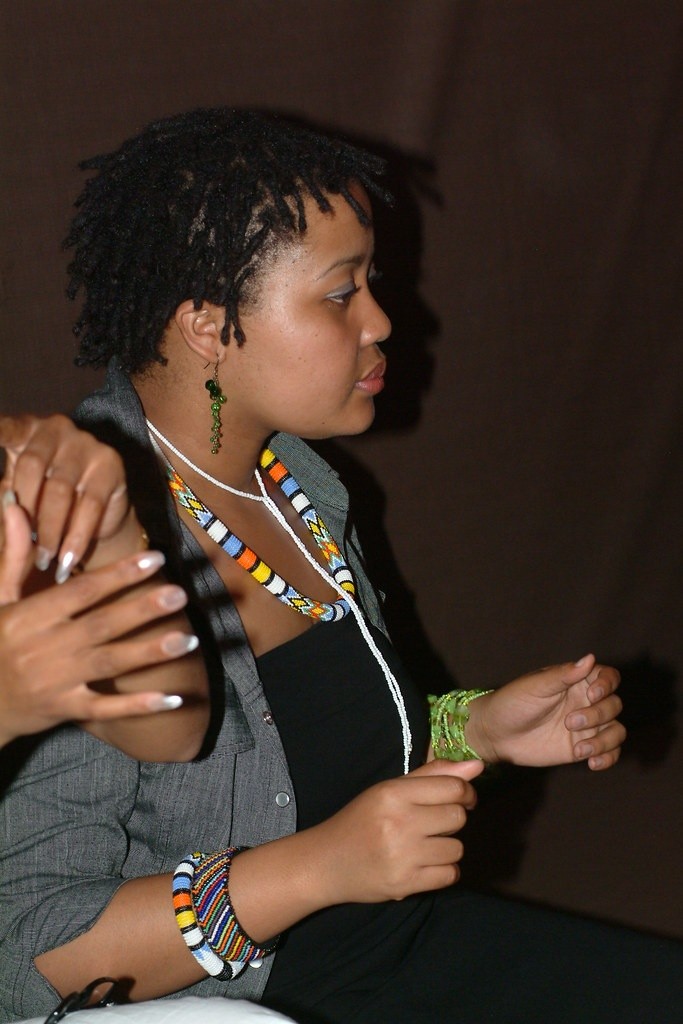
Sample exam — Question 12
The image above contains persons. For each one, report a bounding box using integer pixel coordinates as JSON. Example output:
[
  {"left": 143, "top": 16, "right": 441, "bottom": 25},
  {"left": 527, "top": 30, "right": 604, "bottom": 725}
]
[
  {"left": 0, "top": 110, "right": 683, "bottom": 1024},
  {"left": 0, "top": 410, "right": 214, "bottom": 764}
]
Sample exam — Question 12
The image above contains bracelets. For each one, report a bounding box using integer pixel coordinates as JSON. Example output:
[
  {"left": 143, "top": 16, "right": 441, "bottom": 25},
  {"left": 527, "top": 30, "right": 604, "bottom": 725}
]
[
  {"left": 66, "top": 522, "right": 151, "bottom": 576},
  {"left": 170, "top": 843, "right": 276, "bottom": 980},
  {"left": 420, "top": 685, "right": 492, "bottom": 767}
]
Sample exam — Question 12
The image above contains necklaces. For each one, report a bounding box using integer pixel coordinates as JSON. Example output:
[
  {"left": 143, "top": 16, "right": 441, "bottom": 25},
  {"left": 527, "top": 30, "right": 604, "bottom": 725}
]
[
  {"left": 138, "top": 416, "right": 354, "bottom": 623},
  {"left": 146, "top": 420, "right": 414, "bottom": 775}
]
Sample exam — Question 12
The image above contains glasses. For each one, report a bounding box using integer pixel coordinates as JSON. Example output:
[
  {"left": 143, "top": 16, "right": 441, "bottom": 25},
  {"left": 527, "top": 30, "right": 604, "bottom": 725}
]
[{"left": 43, "top": 977, "right": 120, "bottom": 1024}]
